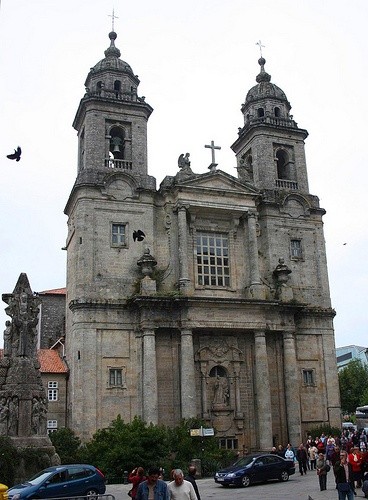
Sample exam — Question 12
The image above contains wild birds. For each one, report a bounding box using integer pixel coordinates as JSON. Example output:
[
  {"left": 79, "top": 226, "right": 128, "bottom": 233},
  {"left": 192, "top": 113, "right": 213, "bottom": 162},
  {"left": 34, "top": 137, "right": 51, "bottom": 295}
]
[
  {"left": 133, "top": 229, "right": 145, "bottom": 243},
  {"left": 343, "top": 243, "right": 347, "bottom": 246},
  {"left": 7, "top": 147, "right": 22, "bottom": 162}
]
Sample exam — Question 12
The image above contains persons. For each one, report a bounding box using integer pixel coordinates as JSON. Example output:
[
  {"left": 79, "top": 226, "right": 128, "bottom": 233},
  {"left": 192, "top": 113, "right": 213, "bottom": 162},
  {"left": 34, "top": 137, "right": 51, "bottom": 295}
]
[
  {"left": 214, "top": 376, "right": 229, "bottom": 405},
  {"left": 178, "top": 152, "right": 191, "bottom": 173},
  {"left": 269, "top": 428, "right": 368, "bottom": 500},
  {"left": 128, "top": 461, "right": 201, "bottom": 500}
]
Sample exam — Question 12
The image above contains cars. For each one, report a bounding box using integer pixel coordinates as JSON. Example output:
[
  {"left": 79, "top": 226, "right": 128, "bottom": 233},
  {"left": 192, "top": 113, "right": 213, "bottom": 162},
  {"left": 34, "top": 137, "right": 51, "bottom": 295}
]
[
  {"left": 5, "top": 465, "right": 107, "bottom": 500},
  {"left": 214, "top": 452, "right": 296, "bottom": 487}
]
